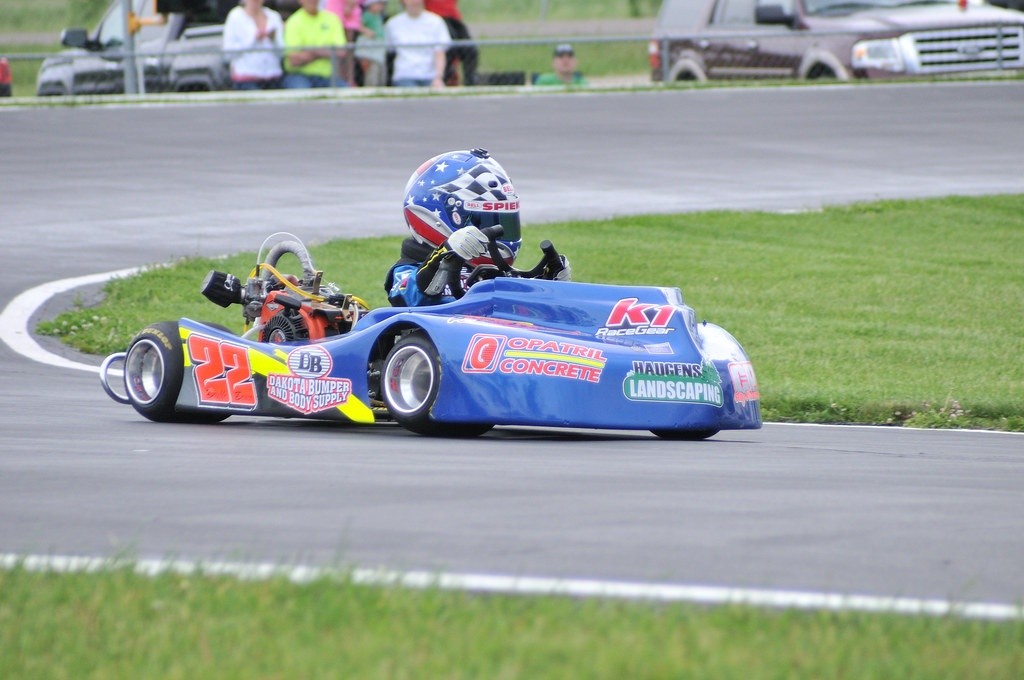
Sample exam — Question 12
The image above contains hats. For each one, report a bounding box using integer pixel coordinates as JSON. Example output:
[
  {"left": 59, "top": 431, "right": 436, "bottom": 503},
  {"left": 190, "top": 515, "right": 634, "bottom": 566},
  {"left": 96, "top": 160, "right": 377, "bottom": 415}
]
[{"left": 554, "top": 44, "right": 574, "bottom": 56}]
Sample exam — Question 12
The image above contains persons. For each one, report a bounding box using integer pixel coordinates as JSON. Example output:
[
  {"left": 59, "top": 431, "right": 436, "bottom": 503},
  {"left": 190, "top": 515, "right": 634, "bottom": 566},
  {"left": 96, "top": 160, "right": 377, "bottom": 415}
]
[
  {"left": 324, "top": 0, "right": 480, "bottom": 87},
  {"left": 278, "top": 0, "right": 349, "bottom": 88},
  {"left": 385, "top": 149, "right": 572, "bottom": 307},
  {"left": 535, "top": 44, "right": 588, "bottom": 86},
  {"left": 223, "top": 0, "right": 285, "bottom": 90}
]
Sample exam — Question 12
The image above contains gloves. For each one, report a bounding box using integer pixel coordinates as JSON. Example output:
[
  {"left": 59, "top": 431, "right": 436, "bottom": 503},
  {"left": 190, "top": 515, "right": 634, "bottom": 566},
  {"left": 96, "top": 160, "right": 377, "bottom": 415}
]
[
  {"left": 553, "top": 254, "right": 571, "bottom": 282},
  {"left": 445, "top": 225, "right": 488, "bottom": 260}
]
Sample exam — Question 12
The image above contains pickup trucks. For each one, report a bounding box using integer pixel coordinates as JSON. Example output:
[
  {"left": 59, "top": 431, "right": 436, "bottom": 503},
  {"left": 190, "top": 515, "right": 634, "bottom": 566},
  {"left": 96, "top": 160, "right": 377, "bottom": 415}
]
[{"left": 35, "top": 1, "right": 289, "bottom": 96}]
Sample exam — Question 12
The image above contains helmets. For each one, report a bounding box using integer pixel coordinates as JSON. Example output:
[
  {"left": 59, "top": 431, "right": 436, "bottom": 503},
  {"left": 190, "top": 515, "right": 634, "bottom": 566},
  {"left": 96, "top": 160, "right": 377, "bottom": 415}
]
[{"left": 403, "top": 148, "right": 523, "bottom": 269}]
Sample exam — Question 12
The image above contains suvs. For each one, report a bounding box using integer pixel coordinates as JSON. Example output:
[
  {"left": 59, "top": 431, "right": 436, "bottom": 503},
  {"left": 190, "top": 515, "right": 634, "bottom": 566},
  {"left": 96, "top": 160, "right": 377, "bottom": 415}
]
[{"left": 648, "top": 0, "right": 1024, "bottom": 85}]
